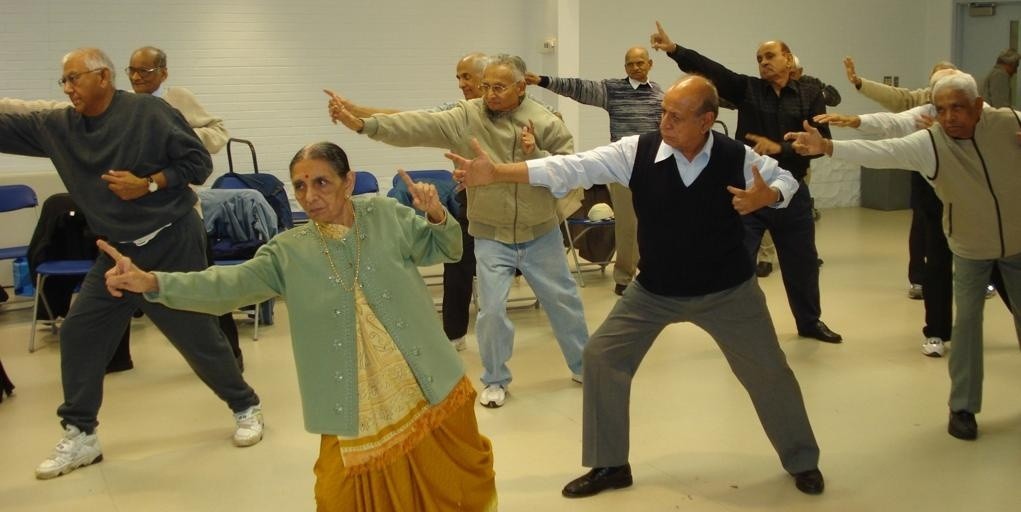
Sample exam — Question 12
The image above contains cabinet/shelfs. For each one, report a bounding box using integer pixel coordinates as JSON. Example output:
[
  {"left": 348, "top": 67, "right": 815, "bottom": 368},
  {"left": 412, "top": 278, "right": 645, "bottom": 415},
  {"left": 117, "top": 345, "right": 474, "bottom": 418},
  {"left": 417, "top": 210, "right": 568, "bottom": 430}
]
[{"left": 860, "top": 165, "right": 915, "bottom": 211}]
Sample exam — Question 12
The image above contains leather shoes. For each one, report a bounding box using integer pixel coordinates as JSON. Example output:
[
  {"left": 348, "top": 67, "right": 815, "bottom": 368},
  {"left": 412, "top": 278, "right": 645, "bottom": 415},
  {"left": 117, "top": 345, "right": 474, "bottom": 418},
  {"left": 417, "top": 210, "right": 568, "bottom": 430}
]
[
  {"left": 797, "top": 318, "right": 842, "bottom": 344},
  {"left": 818, "top": 257, "right": 824, "bottom": 267},
  {"left": 562, "top": 460, "right": 634, "bottom": 499},
  {"left": 756, "top": 260, "right": 773, "bottom": 278},
  {"left": 614, "top": 284, "right": 634, "bottom": 295},
  {"left": 789, "top": 464, "right": 825, "bottom": 496}
]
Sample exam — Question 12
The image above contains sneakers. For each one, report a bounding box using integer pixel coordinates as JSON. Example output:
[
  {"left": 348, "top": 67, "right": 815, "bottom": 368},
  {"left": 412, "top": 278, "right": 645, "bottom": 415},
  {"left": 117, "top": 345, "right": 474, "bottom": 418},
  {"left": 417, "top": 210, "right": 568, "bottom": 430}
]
[
  {"left": 233, "top": 400, "right": 265, "bottom": 448},
  {"left": 34, "top": 423, "right": 103, "bottom": 480},
  {"left": 984, "top": 284, "right": 997, "bottom": 299},
  {"left": 448, "top": 334, "right": 467, "bottom": 353},
  {"left": 948, "top": 410, "right": 979, "bottom": 441},
  {"left": 571, "top": 367, "right": 584, "bottom": 385},
  {"left": 920, "top": 335, "right": 945, "bottom": 357},
  {"left": 908, "top": 281, "right": 925, "bottom": 299},
  {"left": 480, "top": 378, "right": 507, "bottom": 408}
]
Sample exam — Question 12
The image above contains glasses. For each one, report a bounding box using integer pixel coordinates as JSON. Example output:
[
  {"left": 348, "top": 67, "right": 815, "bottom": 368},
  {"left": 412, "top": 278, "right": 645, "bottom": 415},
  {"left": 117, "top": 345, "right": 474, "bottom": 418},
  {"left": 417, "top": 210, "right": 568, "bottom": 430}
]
[
  {"left": 478, "top": 80, "right": 520, "bottom": 94},
  {"left": 124, "top": 64, "right": 164, "bottom": 77},
  {"left": 58, "top": 68, "right": 101, "bottom": 86}
]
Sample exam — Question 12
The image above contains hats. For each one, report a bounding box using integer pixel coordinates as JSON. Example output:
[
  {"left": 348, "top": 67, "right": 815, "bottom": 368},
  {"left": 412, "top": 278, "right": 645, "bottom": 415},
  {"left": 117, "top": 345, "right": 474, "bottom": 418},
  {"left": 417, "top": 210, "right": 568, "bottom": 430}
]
[{"left": 588, "top": 202, "right": 614, "bottom": 221}]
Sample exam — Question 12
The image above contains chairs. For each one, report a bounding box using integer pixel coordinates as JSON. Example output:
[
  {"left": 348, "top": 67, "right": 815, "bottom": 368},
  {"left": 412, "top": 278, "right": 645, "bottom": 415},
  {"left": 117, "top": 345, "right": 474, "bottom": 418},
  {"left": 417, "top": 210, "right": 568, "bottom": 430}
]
[
  {"left": 291, "top": 211, "right": 311, "bottom": 227},
  {"left": 560, "top": 185, "right": 616, "bottom": 288},
  {"left": 194, "top": 187, "right": 280, "bottom": 342},
  {"left": 0, "top": 185, "right": 39, "bottom": 291},
  {"left": 212, "top": 173, "right": 292, "bottom": 234},
  {"left": 446, "top": 182, "right": 545, "bottom": 317},
  {"left": 386, "top": 168, "right": 464, "bottom": 314},
  {"left": 351, "top": 170, "right": 380, "bottom": 195},
  {"left": 28, "top": 190, "right": 108, "bottom": 355}
]
